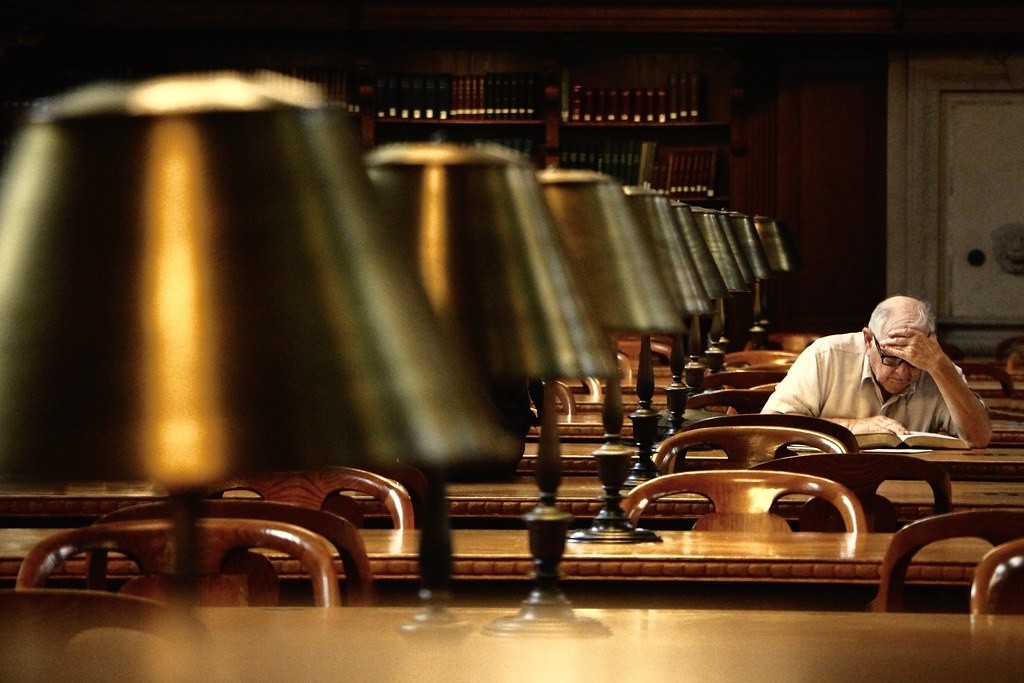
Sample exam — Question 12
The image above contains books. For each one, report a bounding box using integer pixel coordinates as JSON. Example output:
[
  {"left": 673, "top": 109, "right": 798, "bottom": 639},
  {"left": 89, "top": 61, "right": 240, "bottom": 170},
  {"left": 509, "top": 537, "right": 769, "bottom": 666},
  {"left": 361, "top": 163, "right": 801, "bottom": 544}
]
[
  {"left": 556, "top": 139, "right": 724, "bottom": 199},
  {"left": 377, "top": 72, "right": 544, "bottom": 120},
  {"left": 569, "top": 73, "right": 701, "bottom": 121}
]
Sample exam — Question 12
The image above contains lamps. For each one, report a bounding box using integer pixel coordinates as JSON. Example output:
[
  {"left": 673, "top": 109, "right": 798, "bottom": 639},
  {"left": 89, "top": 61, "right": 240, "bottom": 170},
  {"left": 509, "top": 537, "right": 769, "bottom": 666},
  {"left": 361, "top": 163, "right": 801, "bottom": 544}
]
[{"left": 0, "top": 71, "right": 795, "bottom": 605}]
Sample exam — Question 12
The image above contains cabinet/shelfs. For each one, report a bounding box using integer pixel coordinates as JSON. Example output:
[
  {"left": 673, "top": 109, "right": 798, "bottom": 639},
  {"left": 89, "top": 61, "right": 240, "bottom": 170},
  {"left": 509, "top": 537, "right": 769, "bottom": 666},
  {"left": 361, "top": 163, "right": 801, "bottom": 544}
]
[{"left": 75, "top": 6, "right": 899, "bottom": 335}]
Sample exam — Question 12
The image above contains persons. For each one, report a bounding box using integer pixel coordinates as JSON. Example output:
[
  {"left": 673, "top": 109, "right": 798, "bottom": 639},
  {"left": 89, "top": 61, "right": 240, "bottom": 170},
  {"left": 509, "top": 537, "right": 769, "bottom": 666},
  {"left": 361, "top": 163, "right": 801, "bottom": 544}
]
[
  {"left": 756, "top": 294, "right": 992, "bottom": 449},
  {"left": 1006, "top": 339, "right": 1024, "bottom": 376}
]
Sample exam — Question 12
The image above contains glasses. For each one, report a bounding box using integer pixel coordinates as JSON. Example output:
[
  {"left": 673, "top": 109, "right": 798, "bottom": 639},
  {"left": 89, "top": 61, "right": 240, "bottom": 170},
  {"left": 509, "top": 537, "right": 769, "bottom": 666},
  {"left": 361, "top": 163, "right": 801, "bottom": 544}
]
[{"left": 873, "top": 333, "right": 917, "bottom": 369}]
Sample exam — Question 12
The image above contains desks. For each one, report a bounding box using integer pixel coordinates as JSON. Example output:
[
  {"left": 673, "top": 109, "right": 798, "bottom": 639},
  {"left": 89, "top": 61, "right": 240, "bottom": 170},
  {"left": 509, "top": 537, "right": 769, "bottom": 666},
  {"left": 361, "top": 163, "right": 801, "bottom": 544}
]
[{"left": 1, "top": 366, "right": 1024, "bottom": 683}]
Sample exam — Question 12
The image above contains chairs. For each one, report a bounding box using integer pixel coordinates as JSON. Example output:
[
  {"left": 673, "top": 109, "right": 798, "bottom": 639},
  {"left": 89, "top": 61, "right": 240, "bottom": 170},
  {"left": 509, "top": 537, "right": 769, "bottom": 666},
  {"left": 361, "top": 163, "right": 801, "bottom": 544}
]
[{"left": 0, "top": 335, "right": 1024, "bottom": 611}]
